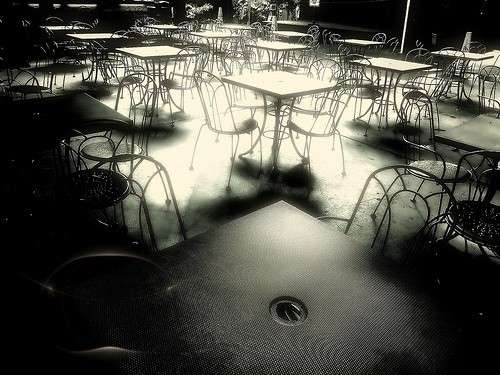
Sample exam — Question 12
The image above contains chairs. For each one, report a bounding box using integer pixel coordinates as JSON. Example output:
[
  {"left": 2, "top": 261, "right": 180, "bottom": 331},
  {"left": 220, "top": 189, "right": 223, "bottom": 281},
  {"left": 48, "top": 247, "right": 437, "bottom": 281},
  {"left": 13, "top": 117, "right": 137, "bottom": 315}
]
[{"left": 2, "top": 9, "right": 499, "bottom": 323}]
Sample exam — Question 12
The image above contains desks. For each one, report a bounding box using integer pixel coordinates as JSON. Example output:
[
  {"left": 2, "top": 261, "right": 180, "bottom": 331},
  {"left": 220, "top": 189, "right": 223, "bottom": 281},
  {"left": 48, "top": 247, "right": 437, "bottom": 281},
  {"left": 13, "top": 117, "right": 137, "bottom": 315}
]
[{"left": 0, "top": 200, "right": 500, "bottom": 375}]
